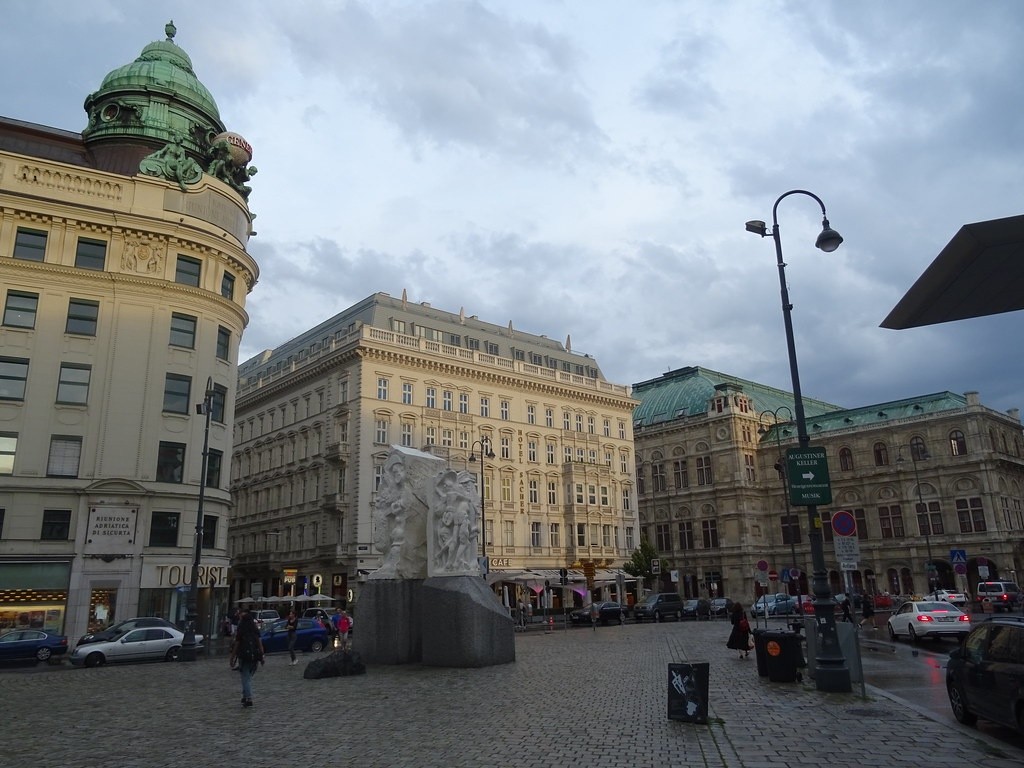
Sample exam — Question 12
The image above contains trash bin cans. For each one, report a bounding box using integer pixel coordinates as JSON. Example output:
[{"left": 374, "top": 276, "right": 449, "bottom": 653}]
[
  {"left": 762, "top": 630, "right": 803, "bottom": 682},
  {"left": 753, "top": 628, "right": 774, "bottom": 676}
]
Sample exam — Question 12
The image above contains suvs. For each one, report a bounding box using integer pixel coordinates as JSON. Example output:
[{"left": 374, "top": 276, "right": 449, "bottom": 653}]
[{"left": 946, "top": 616, "right": 1024, "bottom": 734}]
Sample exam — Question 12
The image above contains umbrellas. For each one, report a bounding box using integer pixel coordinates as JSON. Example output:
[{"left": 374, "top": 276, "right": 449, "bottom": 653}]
[{"left": 234, "top": 594, "right": 336, "bottom": 607}]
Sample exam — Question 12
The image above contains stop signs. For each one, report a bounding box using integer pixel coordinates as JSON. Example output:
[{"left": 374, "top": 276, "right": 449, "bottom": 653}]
[{"left": 768, "top": 570, "right": 777, "bottom": 581}]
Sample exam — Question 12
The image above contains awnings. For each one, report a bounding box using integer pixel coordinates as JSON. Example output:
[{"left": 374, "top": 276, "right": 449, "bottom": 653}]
[{"left": 483, "top": 569, "right": 647, "bottom": 584}]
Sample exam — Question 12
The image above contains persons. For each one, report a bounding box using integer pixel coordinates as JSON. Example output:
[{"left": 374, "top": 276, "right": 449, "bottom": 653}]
[
  {"left": 961, "top": 588, "right": 969, "bottom": 606},
  {"left": 230, "top": 609, "right": 266, "bottom": 706},
  {"left": 877, "top": 589, "right": 890, "bottom": 596},
  {"left": 841, "top": 593, "right": 855, "bottom": 623},
  {"left": 908, "top": 589, "right": 914, "bottom": 595},
  {"left": 433, "top": 469, "right": 480, "bottom": 570},
  {"left": 331, "top": 607, "right": 349, "bottom": 650},
  {"left": 226, "top": 608, "right": 241, "bottom": 641},
  {"left": 726, "top": 602, "right": 753, "bottom": 658},
  {"left": 518, "top": 599, "right": 527, "bottom": 627},
  {"left": 858, "top": 594, "right": 879, "bottom": 630},
  {"left": 285, "top": 609, "right": 299, "bottom": 666}
]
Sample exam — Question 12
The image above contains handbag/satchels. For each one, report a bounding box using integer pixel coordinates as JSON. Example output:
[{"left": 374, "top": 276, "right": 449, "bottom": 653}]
[
  {"left": 740, "top": 619, "right": 748, "bottom": 631},
  {"left": 748, "top": 633, "right": 754, "bottom": 647}
]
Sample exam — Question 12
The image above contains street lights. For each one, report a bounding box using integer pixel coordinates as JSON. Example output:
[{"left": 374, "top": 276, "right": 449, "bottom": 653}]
[
  {"left": 741, "top": 187, "right": 853, "bottom": 692},
  {"left": 637, "top": 456, "right": 664, "bottom": 593},
  {"left": 754, "top": 406, "right": 801, "bottom": 603},
  {"left": 892, "top": 442, "right": 940, "bottom": 601},
  {"left": 467, "top": 434, "right": 495, "bottom": 579},
  {"left": 174, "top": 371, "right": 216, "bottom": 664}
]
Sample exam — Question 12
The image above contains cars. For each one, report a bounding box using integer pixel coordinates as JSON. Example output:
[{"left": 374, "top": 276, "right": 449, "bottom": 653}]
[
  {"left": 792, "top": 595, "right": 814, "bottom": 613},
  {"left": 831, "top": 592, "right": 869, "bottom": 612},
  {"left": 300, "top": 605, "right": 354, "bottom": 631},
  {"left": 710, "top": 598, "right": 734, "bottom": 615},
  {"left": 683, "top": 600, "right": 711, "bottom": 617},
  {"left": 257, "top": 619, "right": 328, "bottom": 652},
  {"left": 570, "top": 600, "right": 630, "bottom": 626},
  {"left": 69, "top": 625, "right": 205, "bottom": 667},
  {"left": 921, "top": 589, "right": 967, "bottom": 606},
  {"left": 77, "top": 616, "right": 188, "bottom": 646},
  {"left": 0, "top": 629, "right": 68, "bottom": 665},
  {"left": 887, "top": 599, "right": 971, "bottom": 644},
  {"left": 751, "top": 592, "right": 797, "bottom": 617}
]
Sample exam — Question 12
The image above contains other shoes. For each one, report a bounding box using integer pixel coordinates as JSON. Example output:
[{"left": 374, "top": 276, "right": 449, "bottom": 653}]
[
  {"left": 745, "top": 654, "right": 748, "bottom": 656},
  {"left": 740, "top": 655, "right": 743, "bottom": 658},
  {"left": 293, "top": 659, "right": 298, "bottom": 666},
  {"left": 873, "top": 627, "right": 877, "bottom": 629},
  {"left": 240, "top": 697, "right": 253, "bottom": 706},
  {"left": 857, "top": 623, "right": 863, "bottom": 629},
  {"left": 289, "top": 662, "right": 293, "bottom": 666}
]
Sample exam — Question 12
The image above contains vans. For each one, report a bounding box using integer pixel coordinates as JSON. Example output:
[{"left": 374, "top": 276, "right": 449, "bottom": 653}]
[
  {"left": 633, "top": 592, "right": 685, "bottom": 620},
  {"left": 976, "top": 581, "right": 1024, "bottom": 613},
  {"left": 248, "top": 610, "right": 279, "bottom": 631}
]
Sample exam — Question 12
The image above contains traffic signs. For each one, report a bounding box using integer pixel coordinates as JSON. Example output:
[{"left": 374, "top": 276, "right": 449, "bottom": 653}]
[{"left": 785, "top": 448, "right": 832, "bottom": 506}]
